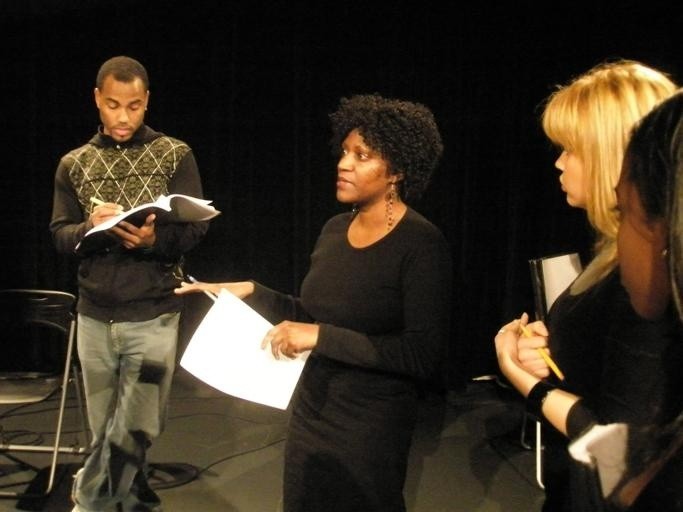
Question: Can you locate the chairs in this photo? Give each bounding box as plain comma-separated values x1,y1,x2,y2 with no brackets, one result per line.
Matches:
0,290,92,498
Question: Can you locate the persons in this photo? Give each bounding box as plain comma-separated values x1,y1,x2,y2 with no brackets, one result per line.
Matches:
561,87,681,510
172,92,452,512
46,56,209,511
493,56,681,511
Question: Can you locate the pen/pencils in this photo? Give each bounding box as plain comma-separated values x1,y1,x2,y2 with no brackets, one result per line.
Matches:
520,324,565,382
187,274,216,303
90,196,124,215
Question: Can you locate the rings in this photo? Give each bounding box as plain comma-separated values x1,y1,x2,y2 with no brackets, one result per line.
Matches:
498,327,504,334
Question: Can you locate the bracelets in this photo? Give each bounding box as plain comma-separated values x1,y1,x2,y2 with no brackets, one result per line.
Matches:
526,380,553,419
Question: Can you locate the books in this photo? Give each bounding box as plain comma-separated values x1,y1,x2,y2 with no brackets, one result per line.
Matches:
71,193,224,260
529,251,584,320
